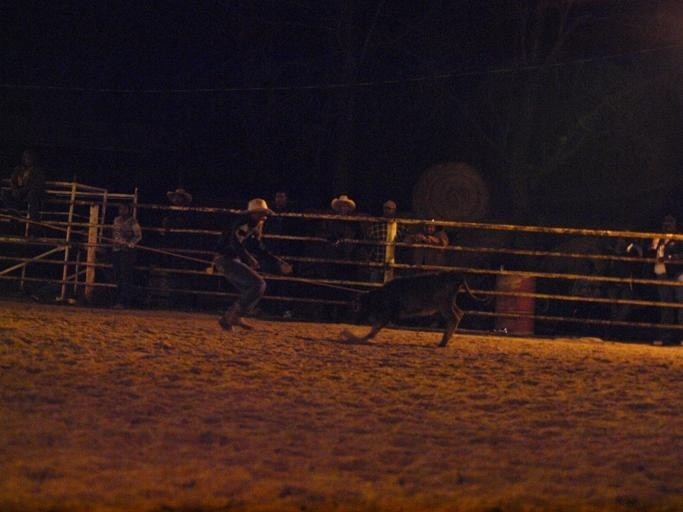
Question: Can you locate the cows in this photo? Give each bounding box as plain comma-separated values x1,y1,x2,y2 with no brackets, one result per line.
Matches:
337,267,496,347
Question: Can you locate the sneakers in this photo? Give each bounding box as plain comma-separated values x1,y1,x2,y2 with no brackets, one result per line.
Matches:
217,315,255,333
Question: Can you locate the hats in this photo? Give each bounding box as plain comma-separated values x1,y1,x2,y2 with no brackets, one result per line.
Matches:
239,198,275,217
330,194,357,214
166,188,192,206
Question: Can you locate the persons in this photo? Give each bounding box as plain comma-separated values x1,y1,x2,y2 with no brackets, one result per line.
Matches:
9,149,46,233
542,214,682,347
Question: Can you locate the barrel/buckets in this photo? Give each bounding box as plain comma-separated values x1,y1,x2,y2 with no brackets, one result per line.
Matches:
496,273,536,335
